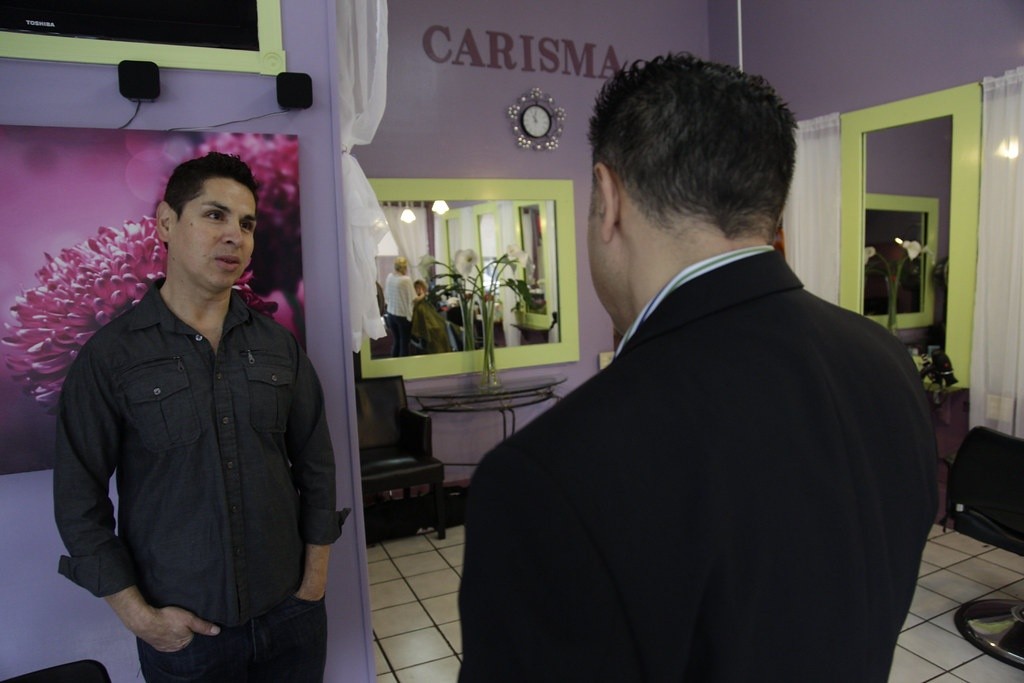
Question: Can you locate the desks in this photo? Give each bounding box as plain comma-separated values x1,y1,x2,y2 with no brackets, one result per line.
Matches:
406,374,568,467
928,386,967,533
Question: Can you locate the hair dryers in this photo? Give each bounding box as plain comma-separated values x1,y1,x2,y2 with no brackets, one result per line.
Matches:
918,345,958,389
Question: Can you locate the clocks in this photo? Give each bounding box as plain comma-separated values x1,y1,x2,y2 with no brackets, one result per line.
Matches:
507,88,566,151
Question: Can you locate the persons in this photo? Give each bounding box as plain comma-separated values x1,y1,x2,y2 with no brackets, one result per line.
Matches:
383,256,452,357
52,150,350,683
457,55,941,683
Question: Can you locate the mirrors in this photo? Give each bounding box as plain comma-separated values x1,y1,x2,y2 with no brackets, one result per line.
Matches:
362,176,580,376
471,204,503,320
838,84,982,388
512,199,550,329
863,193,939,330
443,208,463,285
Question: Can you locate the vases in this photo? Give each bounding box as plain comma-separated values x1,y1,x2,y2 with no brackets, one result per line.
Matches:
458,294,476,351
885,276,901,335
478,297,499,388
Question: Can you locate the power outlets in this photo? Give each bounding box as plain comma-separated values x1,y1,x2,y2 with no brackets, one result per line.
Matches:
598,351,615,370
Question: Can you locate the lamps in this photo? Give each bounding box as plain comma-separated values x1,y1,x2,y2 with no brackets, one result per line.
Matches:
400,200,416,223
431,200,449,214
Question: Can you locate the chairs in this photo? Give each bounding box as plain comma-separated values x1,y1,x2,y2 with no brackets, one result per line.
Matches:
355,375,446,550
942,425,1024,669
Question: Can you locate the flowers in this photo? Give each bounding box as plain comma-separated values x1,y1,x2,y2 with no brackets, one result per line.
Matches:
456,246,545,309
416,255,489,310
888,237,925,283
